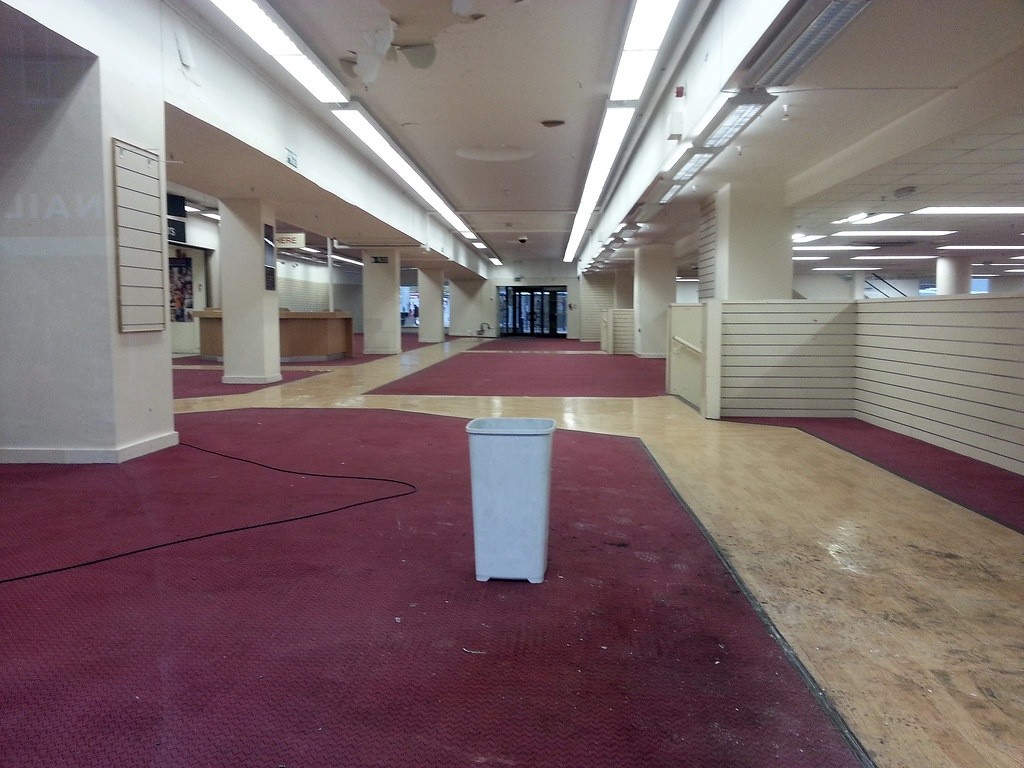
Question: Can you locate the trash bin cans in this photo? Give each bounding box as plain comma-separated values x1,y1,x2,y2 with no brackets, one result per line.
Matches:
465,417,558,584
